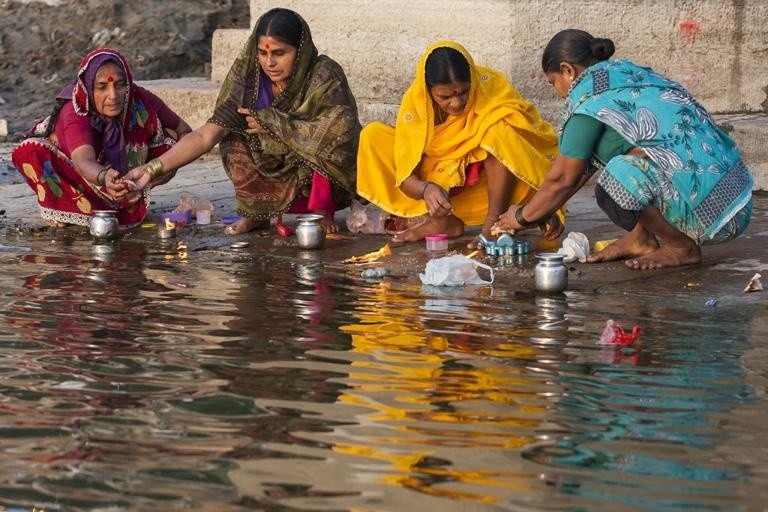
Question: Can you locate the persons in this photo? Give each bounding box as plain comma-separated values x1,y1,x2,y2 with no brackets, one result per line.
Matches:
171,238,358,503
3,233,166,494
358,45,565,251
511,284,764,500
11,47,190,227
491,30,751,269
334,244,545,510
116,8,365,234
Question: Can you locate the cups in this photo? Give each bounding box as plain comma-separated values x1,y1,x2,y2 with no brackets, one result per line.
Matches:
424,233,449,251
196,210,210,224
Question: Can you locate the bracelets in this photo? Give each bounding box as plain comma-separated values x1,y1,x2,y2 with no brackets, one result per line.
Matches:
416,180,432,199
96,165,112,187
144,158,162,176
514,205,539,226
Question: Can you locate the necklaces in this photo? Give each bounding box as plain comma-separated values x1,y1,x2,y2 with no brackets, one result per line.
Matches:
276,82,284,92
436,105,444,125
